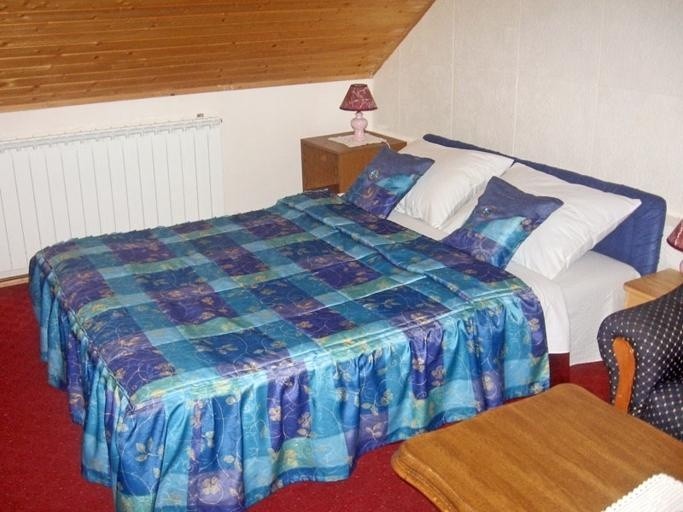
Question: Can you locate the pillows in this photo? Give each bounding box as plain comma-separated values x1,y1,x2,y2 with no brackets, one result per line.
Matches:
439,176,564,269
340,146,434,220
439,160,641,281
394,136,514,229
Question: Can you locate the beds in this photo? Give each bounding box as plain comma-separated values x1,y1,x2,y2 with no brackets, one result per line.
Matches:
30,134,667,512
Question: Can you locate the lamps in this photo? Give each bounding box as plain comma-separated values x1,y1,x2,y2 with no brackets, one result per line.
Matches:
340,84,377,139
667,218,683,271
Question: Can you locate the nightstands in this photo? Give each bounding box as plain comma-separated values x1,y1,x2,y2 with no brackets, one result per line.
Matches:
301,131,407,194
625,269,683,307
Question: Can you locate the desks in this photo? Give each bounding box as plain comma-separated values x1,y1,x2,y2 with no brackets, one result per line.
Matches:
392,382,682,511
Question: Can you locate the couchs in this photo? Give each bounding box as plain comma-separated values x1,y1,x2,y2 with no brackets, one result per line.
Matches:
596,283,682,440
